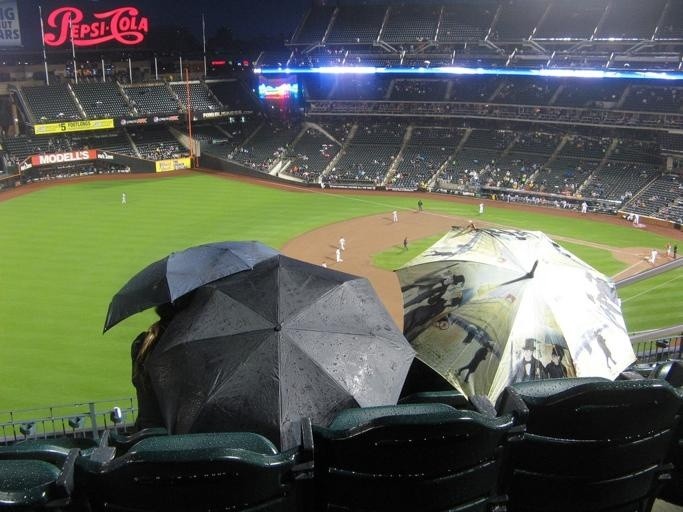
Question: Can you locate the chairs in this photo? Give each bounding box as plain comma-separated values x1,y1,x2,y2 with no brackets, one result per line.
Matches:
0,355,683,512
0,0,683,231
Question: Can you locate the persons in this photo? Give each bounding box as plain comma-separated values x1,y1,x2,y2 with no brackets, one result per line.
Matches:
650,249,657,265
664,240,671,256
424,60,432,67
673,245,678,258
287,117,467,144
392,198,424,222
65,59,114,77
2,153,19,174
95,100,103,105
630,85,680,106
26,134,88,154
350,77,433,95
500,125,652,161
403,237,409,251
121,192,127,208
321,261,327,267
38,162,131,180
130,295,190,428
622,165,683,222
227,144,435,192
310,102,489,116
276,47,362,66
435,157,620,214
512,228,626,381
336,248,342,263
208,104,216,110
480,80,555,100
338,236,345,250
532,107,676,125
127,131,183,160
39,111,154,120
128,99,141,108
401,273,494,384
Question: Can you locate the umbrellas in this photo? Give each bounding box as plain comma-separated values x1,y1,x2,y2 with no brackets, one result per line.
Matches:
103,240,280,336
143,254,417,453
393,223,638,399
